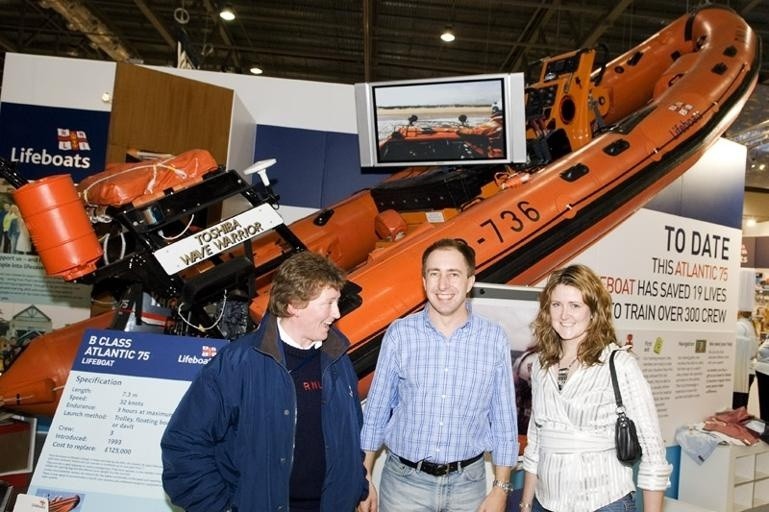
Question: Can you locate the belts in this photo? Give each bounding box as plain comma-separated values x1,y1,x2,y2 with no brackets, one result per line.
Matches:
398,452,485,477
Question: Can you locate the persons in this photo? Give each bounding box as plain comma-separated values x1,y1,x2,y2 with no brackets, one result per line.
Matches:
352,238,522,512
738,311,768,442
519,266,674,512
159,251,369,512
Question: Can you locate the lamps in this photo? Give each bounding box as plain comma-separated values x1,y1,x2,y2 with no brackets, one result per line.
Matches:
219,2,235,21
440,18,456,42
249,58,263,75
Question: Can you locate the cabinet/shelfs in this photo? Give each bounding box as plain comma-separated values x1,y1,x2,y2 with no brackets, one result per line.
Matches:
678,439,769,511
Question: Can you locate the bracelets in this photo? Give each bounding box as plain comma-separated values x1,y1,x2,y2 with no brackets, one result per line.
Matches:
520,501,534,510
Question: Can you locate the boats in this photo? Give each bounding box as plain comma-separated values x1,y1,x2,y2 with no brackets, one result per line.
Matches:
0,5,763,415
379,115,490,160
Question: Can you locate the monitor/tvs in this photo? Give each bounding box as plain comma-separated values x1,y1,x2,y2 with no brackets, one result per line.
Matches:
354,72,527,174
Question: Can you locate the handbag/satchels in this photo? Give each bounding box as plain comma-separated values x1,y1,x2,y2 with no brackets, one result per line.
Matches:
616,412,643,464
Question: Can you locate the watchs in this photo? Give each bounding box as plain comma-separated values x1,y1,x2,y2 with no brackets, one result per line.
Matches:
493,480,515,497
